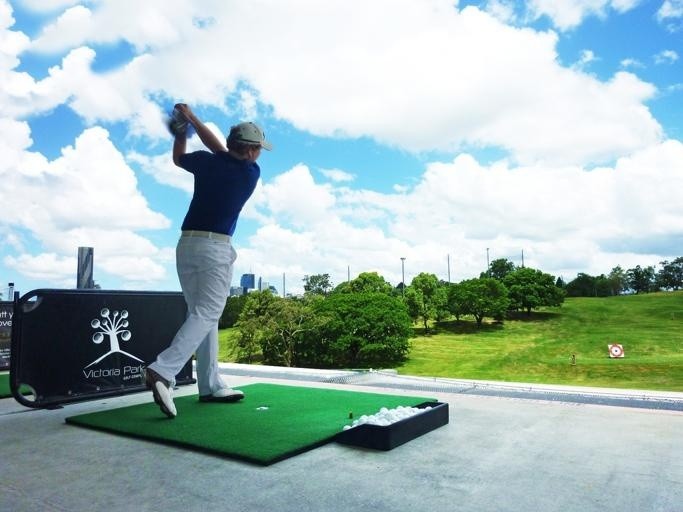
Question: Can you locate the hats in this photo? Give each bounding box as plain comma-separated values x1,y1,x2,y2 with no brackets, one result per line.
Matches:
230,121,272,151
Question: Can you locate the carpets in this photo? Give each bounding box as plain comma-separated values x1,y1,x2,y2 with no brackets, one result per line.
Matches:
65,383,437,467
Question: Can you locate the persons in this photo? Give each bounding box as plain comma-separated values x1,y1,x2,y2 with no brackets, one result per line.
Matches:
144,102,275,418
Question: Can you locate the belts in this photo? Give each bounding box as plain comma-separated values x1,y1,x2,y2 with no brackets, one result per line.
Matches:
181,230,232,244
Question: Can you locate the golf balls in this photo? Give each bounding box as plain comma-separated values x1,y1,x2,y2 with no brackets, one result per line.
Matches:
342,405,432,431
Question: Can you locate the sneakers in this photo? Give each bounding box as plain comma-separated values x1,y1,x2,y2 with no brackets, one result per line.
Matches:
198,385,245,402
139,366,178,420
